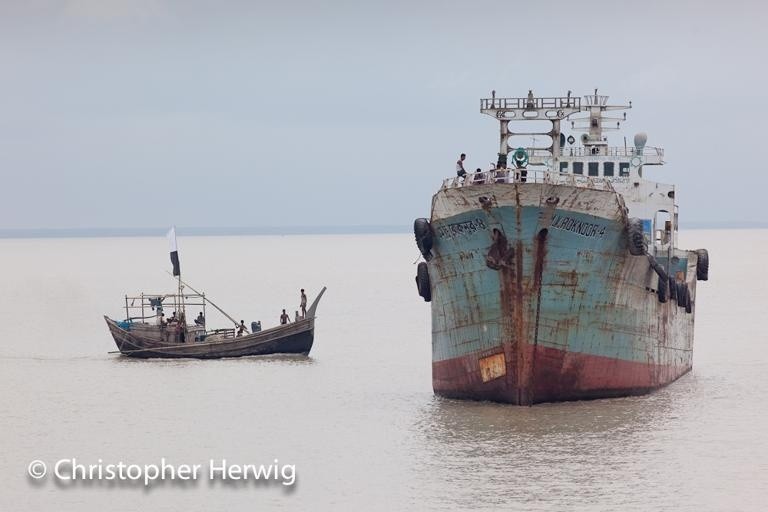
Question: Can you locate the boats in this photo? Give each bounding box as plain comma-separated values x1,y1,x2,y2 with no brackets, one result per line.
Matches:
417,93,713,405
102,286,327,359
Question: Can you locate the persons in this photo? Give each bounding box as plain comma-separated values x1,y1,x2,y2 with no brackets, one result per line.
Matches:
300,289,307,318
159,312,187,342
236,320,247,337
474,168,485,184
512,148,528,181
195,312,204,325
280,309,291,325
457,153,466,178
